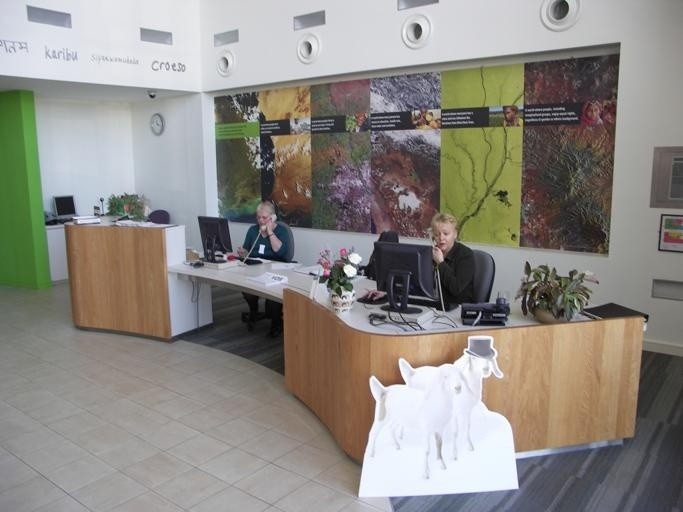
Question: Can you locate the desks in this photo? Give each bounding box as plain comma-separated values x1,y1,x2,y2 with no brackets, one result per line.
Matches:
43,214,116,282
61,214,210,343
168,250,371,306
281,287,647,464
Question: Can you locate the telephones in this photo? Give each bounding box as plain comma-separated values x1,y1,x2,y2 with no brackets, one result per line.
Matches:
260,215,277,232
428,228,435,247
461,292,509,325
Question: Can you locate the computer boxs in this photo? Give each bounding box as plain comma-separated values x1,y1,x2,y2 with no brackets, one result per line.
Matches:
195,257,241,270
370,302,436,326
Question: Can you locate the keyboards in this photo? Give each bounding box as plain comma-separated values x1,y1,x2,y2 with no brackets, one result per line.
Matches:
394,295,438,308
235,257,262,265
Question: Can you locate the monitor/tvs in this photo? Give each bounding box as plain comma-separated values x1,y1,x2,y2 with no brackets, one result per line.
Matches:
373,242,433,314
53,195,77,219
197,216,232,263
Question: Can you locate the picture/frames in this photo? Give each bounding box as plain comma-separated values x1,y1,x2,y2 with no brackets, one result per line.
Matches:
657,214,682,252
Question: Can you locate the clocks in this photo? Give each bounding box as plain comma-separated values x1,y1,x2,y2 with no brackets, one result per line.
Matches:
150,112,165,136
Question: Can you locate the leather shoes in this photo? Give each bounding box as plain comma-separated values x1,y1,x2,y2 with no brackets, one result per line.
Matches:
248,312,258,331
267,319,282,338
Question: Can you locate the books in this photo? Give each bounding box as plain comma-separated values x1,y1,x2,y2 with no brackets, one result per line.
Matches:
71,215,101,225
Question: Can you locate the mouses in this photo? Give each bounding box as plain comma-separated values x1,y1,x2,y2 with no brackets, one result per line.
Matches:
363,293,376,302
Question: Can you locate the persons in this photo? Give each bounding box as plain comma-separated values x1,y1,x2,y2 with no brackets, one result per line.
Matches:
364,213,475,319
290,115,302,135
500,105,523,126
350,114,368,132
410,104,437,129
237,201,294,333
579,99,604,125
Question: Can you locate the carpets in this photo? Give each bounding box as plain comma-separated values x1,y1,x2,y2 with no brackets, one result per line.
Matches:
175,282,683,511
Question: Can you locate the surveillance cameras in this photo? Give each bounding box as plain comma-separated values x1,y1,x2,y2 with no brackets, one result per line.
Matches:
148,91,156,98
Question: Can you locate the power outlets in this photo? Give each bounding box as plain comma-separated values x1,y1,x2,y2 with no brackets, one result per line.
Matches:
96,194,104,203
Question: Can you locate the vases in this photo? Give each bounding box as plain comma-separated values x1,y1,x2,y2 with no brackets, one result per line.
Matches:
328,292,354,314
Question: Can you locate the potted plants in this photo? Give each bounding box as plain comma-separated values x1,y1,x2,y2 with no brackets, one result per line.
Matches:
513,260,600,327
107,194,147,221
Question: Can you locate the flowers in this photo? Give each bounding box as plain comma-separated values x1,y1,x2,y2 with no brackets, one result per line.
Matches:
316,247,362,297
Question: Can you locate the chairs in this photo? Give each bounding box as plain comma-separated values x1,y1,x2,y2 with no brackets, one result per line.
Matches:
347,229,399,294
239,220,296,339
435,249,496,313
145,209,170,228
